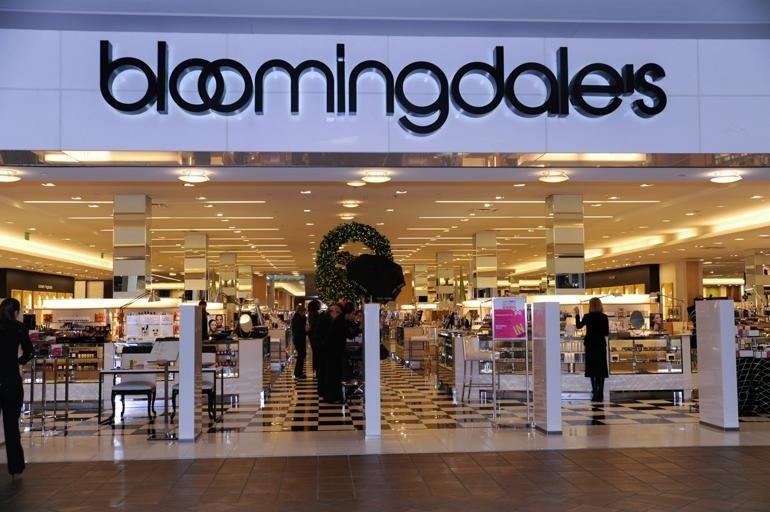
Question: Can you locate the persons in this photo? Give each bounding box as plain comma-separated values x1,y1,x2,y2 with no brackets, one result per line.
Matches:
307,298,364,403
250,309,257,317
199,300,210,340
575,296,611,403
291,304,307,379
209,319,218,334
651,314,662,330
1,297,37,480
215,315,225,333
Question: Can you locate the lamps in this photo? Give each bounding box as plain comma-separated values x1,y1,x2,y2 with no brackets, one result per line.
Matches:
536,167,571,185
361,170,392,184
0,166,21,185
177,167,213,183
708,168,744,185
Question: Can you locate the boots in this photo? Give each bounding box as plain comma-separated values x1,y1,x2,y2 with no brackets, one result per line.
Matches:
591,377,605,402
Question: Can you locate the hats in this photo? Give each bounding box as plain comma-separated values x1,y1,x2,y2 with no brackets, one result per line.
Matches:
330,304,343,313
